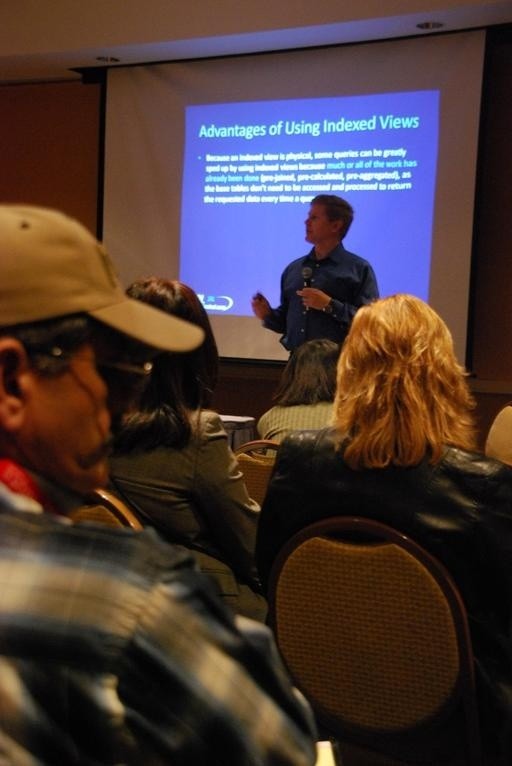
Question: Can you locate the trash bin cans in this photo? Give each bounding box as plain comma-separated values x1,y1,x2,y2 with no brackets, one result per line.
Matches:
322,297,336,314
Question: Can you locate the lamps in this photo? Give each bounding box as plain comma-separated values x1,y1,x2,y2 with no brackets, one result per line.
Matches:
266,516,482,766
235,439,279,504
65,488,151,530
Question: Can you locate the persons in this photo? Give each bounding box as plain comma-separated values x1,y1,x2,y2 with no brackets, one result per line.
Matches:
252,193,381,351
253,288,512,766
107,275,272,626
2,204,319,766
254,338,343,462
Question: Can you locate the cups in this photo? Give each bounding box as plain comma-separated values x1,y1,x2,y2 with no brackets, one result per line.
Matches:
36,348,156,395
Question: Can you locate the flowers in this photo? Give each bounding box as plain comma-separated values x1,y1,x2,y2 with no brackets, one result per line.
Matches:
302,265,314,313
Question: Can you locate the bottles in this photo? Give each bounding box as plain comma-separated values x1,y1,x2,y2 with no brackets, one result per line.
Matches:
0,202,208,354
